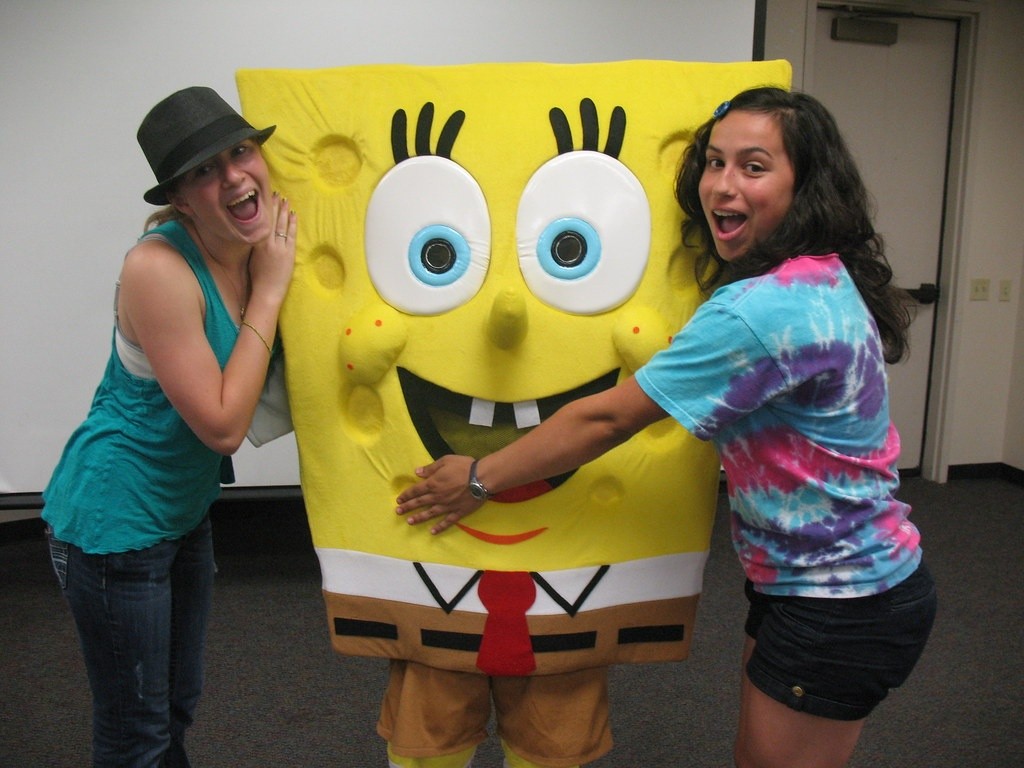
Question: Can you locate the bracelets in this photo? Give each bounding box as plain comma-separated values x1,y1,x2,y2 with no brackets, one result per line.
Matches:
242,321,271,353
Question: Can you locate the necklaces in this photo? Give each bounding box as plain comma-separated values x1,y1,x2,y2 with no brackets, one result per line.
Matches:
217,262,247,317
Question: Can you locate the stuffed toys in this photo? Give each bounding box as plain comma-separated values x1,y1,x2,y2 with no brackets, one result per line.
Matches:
238,59,797,768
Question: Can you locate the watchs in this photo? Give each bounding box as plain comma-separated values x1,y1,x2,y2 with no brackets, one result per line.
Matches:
468,459,493,501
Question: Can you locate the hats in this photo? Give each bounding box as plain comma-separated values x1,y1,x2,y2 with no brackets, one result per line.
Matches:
137,85,277,205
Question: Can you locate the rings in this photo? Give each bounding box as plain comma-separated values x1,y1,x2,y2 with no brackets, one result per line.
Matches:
275,233,286,238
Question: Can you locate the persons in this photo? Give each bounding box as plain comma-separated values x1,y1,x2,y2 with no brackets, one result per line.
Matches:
398,87,939,768
40,87,298,768
